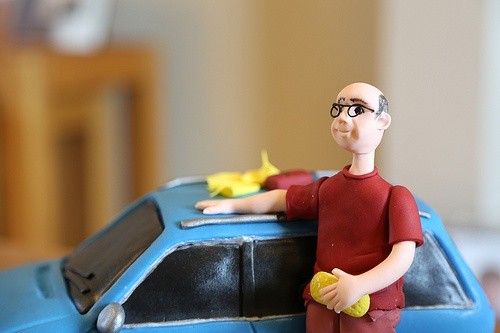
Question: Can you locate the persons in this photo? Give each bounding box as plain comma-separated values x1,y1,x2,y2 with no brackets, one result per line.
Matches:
193,82,423,333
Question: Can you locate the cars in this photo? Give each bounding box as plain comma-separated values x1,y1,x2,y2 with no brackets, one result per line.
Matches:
0,169,497,333
